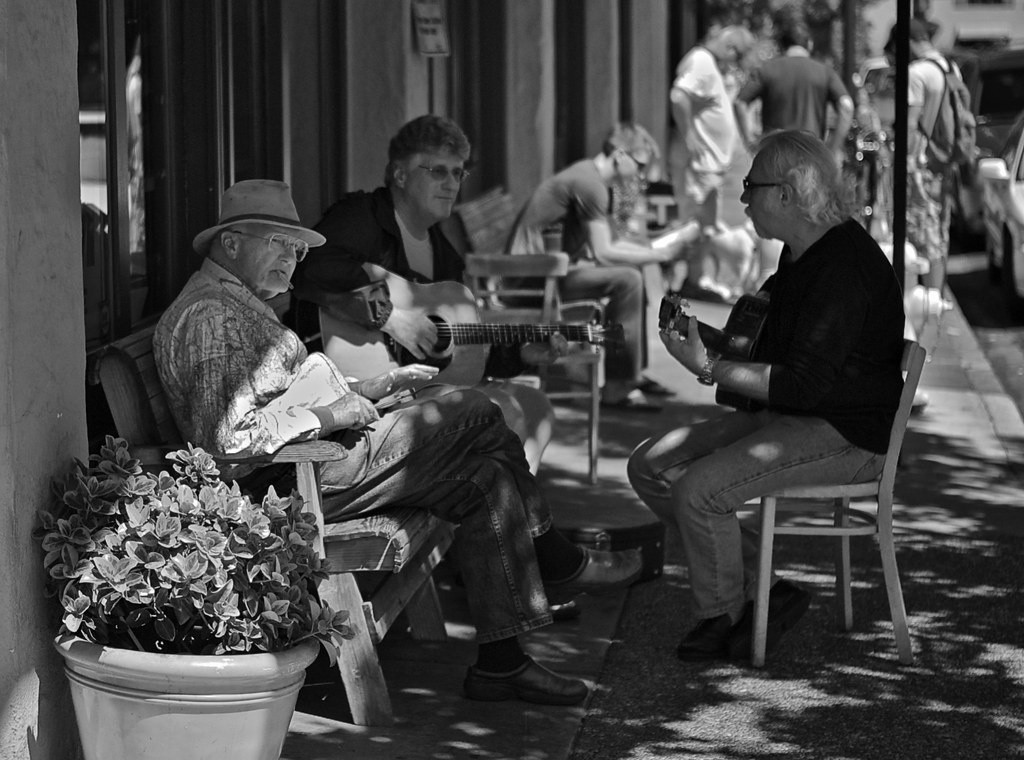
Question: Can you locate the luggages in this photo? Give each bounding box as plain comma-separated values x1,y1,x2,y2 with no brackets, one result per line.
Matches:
733,45,743,61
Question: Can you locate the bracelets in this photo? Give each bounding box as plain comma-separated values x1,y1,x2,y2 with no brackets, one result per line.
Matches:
357,379,380,405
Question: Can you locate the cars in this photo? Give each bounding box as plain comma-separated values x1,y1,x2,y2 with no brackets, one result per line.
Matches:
851,25,1024,300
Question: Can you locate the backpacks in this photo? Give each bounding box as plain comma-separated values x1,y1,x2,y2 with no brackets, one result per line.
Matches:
908,56,977,174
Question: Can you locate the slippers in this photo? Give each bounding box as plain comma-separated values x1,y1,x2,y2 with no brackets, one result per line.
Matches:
636,375,677,397
601,392,663,413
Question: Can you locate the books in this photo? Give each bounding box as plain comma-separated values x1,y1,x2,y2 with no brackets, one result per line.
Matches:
650,219,699,250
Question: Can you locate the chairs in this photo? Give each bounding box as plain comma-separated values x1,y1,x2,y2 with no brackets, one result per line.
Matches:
736,338,925,671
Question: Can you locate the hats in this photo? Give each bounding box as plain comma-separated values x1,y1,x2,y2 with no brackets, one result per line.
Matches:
192,178,327,257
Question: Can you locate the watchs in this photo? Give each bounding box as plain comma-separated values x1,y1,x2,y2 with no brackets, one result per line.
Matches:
697,354,721,386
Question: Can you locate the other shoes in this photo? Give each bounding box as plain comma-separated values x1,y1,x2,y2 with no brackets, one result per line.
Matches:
458,651,589,708
541,545,644,591
676,577,812,663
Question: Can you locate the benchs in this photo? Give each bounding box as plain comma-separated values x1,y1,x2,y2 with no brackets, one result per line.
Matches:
88,187,677,726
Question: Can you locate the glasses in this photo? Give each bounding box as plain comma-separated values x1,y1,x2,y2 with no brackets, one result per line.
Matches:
628,151,646,171
232,230,309,262
418,163,470,185
742,179,782,192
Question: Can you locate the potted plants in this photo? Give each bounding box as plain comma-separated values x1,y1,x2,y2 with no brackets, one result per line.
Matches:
30,435,354,759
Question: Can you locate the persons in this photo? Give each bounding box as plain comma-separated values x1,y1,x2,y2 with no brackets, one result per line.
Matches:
733,17,854,292
502,121,701,418
506,123,700,413
881,20,977,316
627,127,905,668
669,25,741,304
289,117,563,587
153,179,591,704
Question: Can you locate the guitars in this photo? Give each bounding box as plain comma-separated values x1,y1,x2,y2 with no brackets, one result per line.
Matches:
658,268,778,416
319,261,627,410
